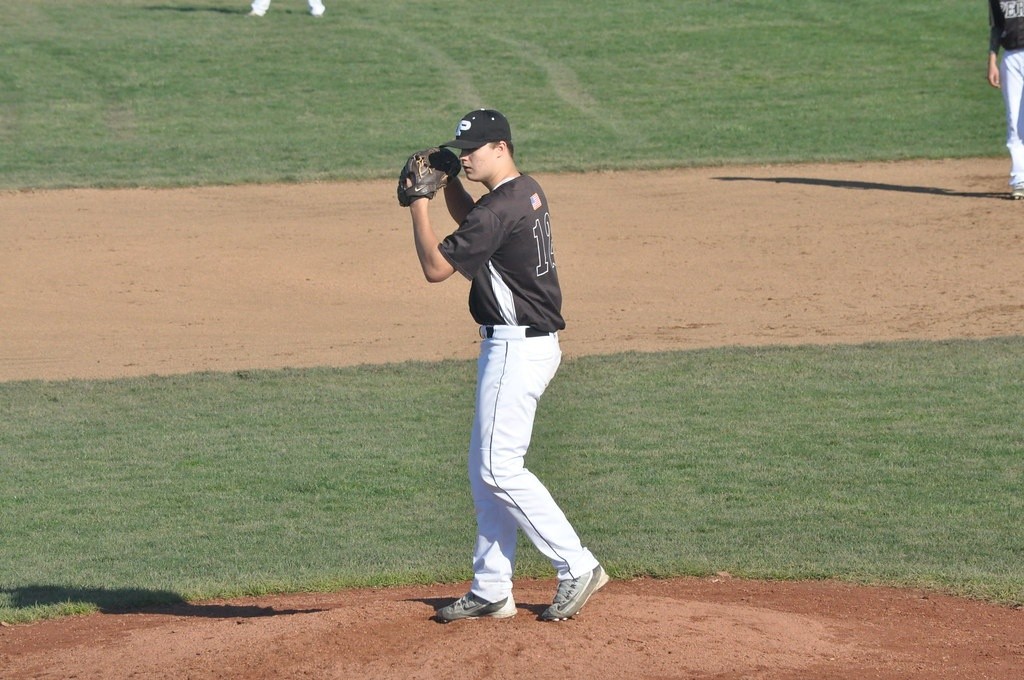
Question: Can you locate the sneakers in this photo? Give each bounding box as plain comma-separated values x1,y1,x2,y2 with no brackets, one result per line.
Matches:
437,591,518,623
542,565,610,621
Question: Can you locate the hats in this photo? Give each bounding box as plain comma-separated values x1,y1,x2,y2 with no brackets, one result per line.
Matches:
438,108,511,149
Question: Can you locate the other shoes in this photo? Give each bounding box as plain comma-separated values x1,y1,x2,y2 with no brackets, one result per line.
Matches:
249,10,258,16
1011,189,1024,200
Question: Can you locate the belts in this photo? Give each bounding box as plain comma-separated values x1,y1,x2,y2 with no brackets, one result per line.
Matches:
479,326,549,339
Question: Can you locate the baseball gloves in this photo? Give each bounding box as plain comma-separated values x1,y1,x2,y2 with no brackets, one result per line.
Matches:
397,146,461,207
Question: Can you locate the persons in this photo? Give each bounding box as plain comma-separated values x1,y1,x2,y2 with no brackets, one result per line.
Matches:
398,110,611,624
987,0,1024,199
249,0,326,18
